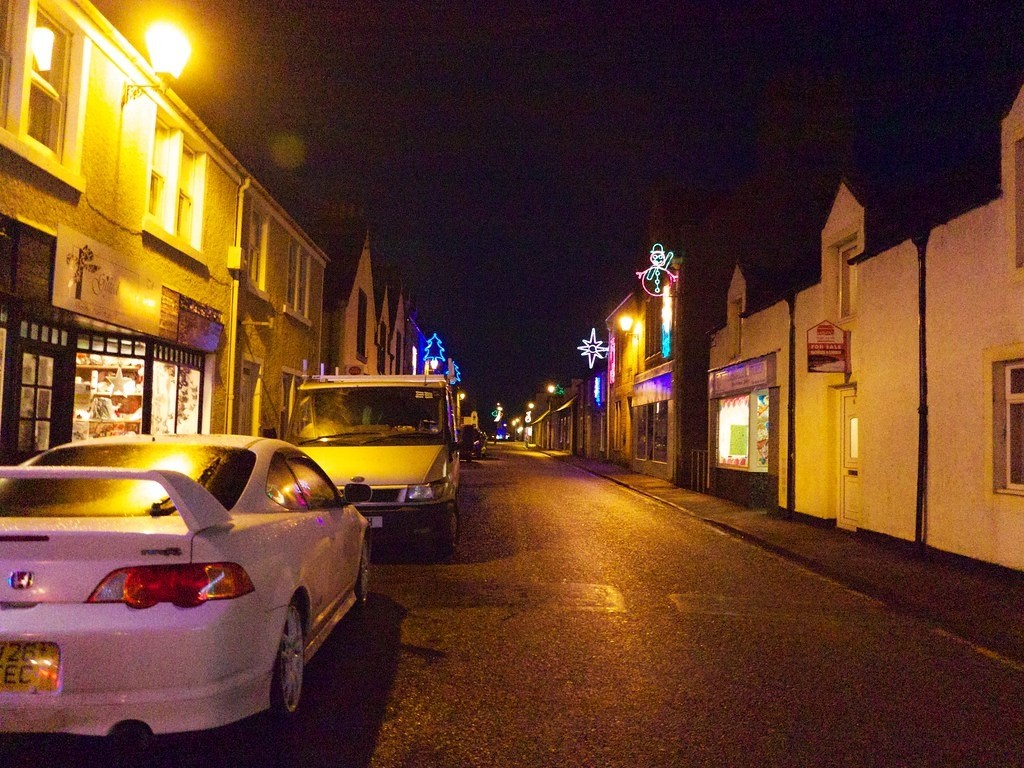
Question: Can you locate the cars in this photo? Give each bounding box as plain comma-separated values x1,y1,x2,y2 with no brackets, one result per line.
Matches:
0,431,371,751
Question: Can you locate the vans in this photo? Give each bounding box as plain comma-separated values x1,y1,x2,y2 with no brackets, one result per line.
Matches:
288,372,460,556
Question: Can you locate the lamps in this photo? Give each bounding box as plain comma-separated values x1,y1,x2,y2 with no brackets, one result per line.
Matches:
121,21,193,107
